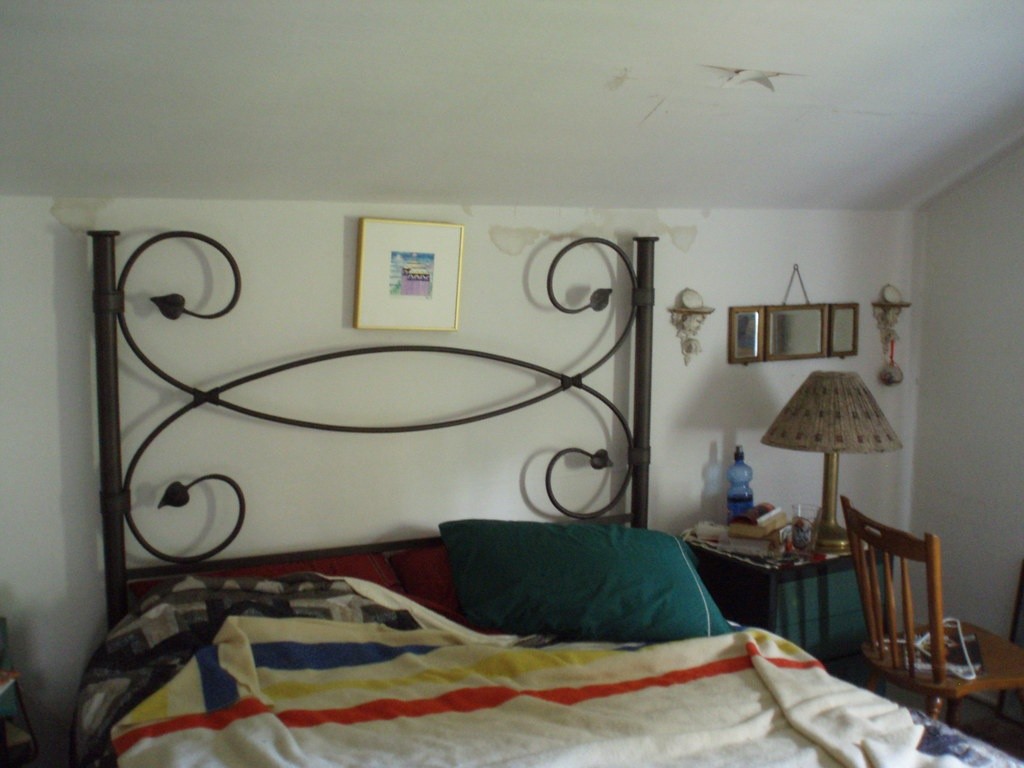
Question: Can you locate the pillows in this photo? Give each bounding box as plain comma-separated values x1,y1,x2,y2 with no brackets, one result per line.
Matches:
129,553,404,600
439,519,732,639
390,542,461,619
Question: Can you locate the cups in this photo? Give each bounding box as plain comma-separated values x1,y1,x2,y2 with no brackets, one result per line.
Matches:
791,503,822,557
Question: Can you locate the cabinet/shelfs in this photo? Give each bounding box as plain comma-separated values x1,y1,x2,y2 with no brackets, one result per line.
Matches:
682,532,893,700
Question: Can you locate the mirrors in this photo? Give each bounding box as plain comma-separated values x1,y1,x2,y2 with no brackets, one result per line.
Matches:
728,302,859,363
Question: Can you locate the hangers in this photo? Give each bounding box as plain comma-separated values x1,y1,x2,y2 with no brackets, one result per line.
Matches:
875,617,976,682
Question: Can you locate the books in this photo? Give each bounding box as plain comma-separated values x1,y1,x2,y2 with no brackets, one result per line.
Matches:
874,631,984,678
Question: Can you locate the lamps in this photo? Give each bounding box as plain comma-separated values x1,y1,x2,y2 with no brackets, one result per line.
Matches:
669,288,716,365
761,371,903,548
872,284,911,384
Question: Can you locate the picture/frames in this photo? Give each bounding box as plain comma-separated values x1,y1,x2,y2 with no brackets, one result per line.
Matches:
354,219,463,332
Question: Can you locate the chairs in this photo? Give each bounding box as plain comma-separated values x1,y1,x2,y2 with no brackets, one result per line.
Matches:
840,496,1024,752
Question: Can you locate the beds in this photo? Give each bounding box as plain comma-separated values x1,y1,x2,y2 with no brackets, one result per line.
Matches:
69,232,1024,768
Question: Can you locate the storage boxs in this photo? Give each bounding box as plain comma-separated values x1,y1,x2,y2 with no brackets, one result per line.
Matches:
729,507,786,540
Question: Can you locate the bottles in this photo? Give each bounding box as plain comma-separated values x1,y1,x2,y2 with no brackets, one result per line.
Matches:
726,444,758,527
